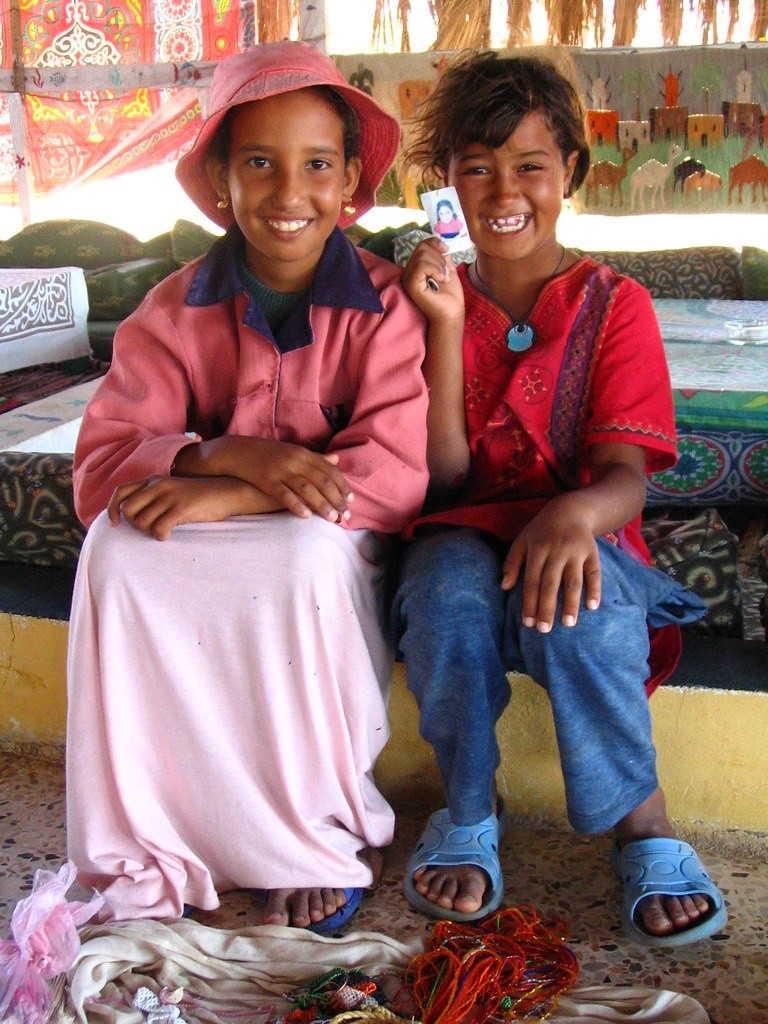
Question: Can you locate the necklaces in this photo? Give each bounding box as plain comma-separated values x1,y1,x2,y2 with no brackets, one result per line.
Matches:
475,244,565,352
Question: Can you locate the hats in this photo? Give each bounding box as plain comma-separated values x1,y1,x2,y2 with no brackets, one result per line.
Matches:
176,46,399,231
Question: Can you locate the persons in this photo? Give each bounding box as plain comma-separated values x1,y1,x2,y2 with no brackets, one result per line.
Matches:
390,49,731,950
434,199,464,239
63,41,430,934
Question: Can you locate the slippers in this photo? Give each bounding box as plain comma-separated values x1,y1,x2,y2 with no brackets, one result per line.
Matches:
404,790,506,920
610,837,727,948
263,874,361,933
